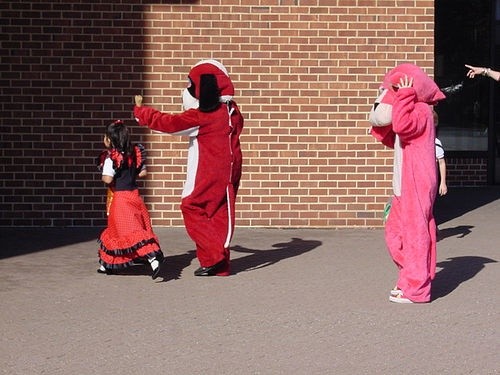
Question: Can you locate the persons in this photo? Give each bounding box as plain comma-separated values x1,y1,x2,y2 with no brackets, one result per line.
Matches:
465,64,500,82
434,137,448,196
97,120,165,280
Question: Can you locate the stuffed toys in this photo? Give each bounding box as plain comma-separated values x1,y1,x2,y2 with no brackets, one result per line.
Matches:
134,59,244,277
367,64,446,304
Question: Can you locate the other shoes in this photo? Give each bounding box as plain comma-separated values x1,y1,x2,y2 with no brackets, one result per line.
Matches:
97,264,113,275
148,253,168,280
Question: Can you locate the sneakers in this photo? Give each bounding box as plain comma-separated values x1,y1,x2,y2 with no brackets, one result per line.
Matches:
388,291,414,304
390,285,402,295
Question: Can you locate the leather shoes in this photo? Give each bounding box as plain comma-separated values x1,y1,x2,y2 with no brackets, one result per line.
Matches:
193,260,227,276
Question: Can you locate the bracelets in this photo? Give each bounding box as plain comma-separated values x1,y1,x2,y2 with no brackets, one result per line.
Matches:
482,67,490,77
